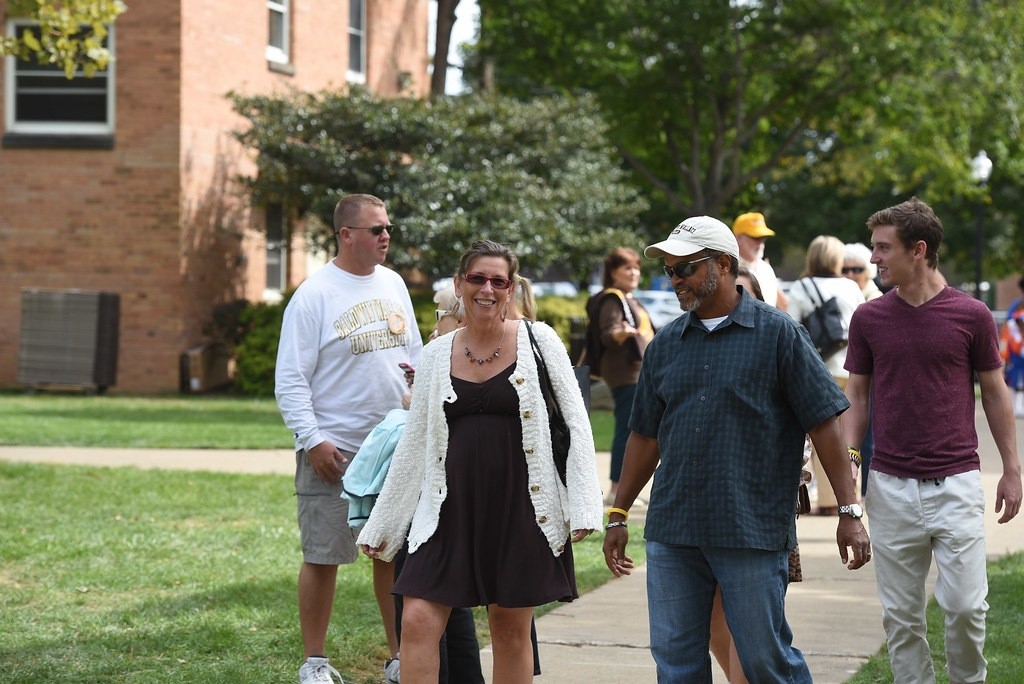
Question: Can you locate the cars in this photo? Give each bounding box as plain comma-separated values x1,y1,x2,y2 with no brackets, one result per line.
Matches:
630,289,683,334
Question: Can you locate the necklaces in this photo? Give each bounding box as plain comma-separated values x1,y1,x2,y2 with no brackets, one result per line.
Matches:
464,326,505,364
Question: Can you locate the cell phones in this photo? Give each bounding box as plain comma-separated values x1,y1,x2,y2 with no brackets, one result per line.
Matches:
398,362,415,383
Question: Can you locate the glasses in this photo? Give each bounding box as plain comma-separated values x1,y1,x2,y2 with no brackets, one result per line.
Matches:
436,310,452,322
842,267,863,276
337,225,395,235
663,255,711,279
464,273,512,289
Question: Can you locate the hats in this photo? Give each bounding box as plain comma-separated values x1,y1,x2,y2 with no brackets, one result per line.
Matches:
644,216,739,262
734,211,776,237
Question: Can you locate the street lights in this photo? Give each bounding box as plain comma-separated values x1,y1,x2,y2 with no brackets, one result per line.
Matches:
969,148,993,384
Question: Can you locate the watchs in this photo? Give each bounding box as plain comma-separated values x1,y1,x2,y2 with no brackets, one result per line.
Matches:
838,503,863,519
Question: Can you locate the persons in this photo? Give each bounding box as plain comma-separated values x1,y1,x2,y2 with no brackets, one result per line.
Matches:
841,197,1023,684
355,241,603,684
586,247,658,506
273,194,424,684
602,216,871,684
999,277,1024,420
732,212,884,517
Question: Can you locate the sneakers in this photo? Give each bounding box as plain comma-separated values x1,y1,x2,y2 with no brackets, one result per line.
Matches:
384,653,401,684
298,656,344,684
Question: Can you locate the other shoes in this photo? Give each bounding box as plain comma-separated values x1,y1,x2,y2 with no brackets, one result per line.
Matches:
820,506,839,515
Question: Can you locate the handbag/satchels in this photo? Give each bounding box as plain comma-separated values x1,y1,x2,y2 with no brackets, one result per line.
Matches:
522,319,591,488
800,275,849,362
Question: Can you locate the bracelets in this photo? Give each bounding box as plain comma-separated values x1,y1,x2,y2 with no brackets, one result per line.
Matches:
606,508,628,521
849,453,860,468
848,450,861,463
605,522,627,530
